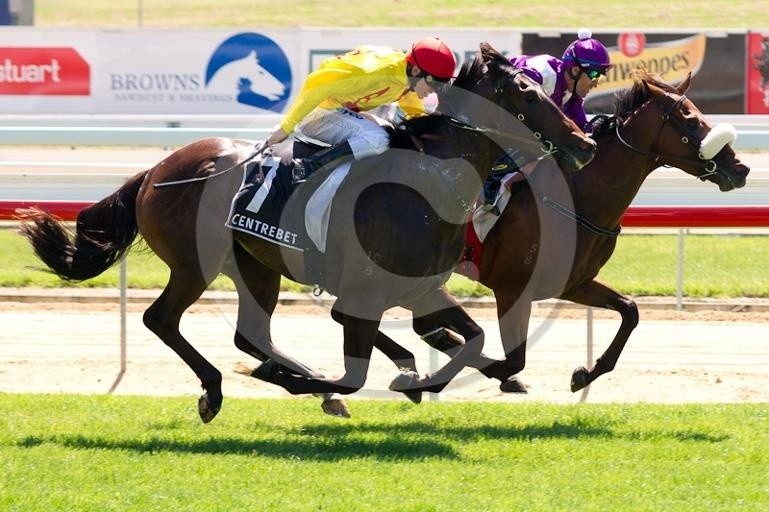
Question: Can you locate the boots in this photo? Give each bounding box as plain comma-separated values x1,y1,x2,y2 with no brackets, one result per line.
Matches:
292,140,354,180
481,146,538,215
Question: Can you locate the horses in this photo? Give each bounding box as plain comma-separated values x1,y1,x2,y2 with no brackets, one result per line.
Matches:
330,67,752,402
14,40,597,425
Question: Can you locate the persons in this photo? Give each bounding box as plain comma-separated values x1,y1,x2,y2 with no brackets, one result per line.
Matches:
258,34,456,186
461,35,615,225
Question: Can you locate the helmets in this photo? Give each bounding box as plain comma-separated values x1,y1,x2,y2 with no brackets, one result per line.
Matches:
407,37,456,82
562,29,615,70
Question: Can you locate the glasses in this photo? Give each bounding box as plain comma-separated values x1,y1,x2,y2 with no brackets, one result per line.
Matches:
572,49,601,82
412,47,444,88
